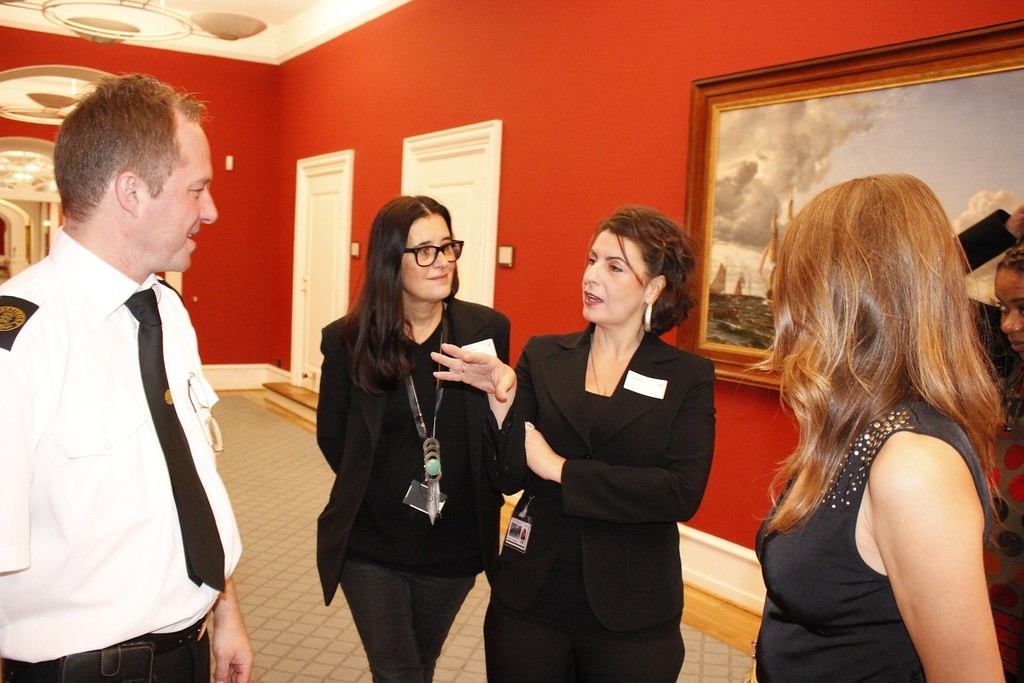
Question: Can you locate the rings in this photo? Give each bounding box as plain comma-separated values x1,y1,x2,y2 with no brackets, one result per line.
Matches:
460,363,464,372
526,421,534,431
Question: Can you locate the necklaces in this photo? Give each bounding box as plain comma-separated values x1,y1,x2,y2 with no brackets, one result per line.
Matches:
589,334,642,397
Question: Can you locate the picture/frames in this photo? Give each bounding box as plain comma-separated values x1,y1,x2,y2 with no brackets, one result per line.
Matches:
677,20,1024,432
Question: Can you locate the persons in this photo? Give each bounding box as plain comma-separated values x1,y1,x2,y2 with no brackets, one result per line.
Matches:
317,197,510,683
431,204,715,682
0,73,256,683
736,173,1005,683
957,204,1024,682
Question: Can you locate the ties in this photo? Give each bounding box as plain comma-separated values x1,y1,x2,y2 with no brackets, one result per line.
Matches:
124,283,228,593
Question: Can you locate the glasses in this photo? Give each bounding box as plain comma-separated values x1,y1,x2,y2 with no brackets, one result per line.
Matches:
401,237,466,267
